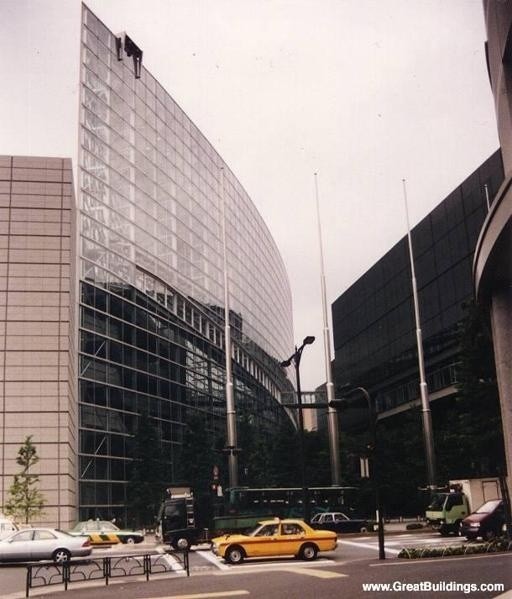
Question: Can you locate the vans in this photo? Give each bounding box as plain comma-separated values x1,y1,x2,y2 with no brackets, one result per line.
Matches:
460,500,507,542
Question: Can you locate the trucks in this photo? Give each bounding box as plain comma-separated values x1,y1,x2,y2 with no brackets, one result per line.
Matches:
426,477,504,537
158,487,361,552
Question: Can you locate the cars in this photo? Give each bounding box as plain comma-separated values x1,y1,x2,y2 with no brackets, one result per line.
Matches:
0,519,143,566
309,512,379,533
210,517,338,563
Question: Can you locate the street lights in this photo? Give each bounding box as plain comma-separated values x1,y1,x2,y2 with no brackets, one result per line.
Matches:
281,336,315,522
